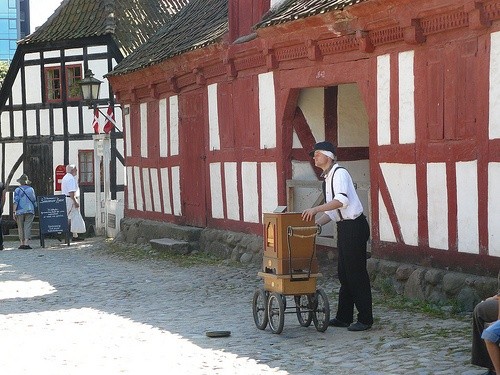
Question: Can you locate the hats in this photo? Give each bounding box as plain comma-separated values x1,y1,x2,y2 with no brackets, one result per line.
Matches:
16,173,32,185
309,141,336,156
66,165,76,172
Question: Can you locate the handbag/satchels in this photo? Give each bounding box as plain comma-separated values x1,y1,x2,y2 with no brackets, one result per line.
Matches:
34,201,39,216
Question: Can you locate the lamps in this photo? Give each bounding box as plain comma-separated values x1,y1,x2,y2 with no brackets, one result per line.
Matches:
77,69,130,117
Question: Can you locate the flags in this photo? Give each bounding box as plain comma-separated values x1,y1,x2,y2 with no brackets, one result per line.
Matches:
103,105,115,134
92,108,99,134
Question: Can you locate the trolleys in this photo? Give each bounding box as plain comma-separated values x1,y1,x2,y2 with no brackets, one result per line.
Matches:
251,206,330,334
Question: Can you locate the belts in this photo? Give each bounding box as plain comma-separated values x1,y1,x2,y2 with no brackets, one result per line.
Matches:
65,195,78,199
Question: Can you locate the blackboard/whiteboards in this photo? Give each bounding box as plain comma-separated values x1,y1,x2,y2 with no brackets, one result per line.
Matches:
37,195,69,234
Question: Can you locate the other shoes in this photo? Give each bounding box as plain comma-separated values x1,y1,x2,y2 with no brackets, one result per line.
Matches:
72,237,84,241
18,245,33,249
322,319,350,327
348,321,371,331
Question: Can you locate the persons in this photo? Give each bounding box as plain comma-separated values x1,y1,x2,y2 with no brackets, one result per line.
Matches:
0,181,6,251
58,164,85,242
13,174,38,249
472,293,500,375
301,141,373,331
